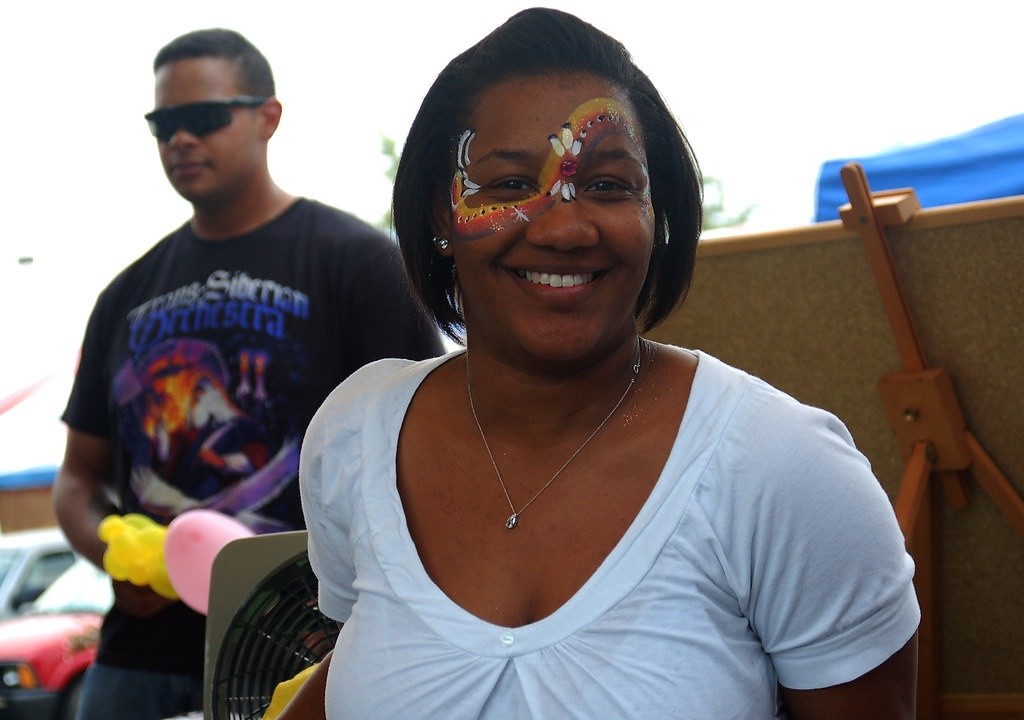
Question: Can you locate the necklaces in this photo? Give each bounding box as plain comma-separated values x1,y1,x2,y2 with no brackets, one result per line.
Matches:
466,336,641,530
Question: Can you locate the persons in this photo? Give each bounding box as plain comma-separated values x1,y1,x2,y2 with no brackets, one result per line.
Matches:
52,28,447,720
277,7,924,719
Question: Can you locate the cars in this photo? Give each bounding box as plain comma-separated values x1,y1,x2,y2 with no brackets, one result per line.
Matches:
0,527,80,633
0,556,116,720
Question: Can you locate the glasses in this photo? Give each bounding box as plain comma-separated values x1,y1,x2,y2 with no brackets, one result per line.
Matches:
144,94,268,142
